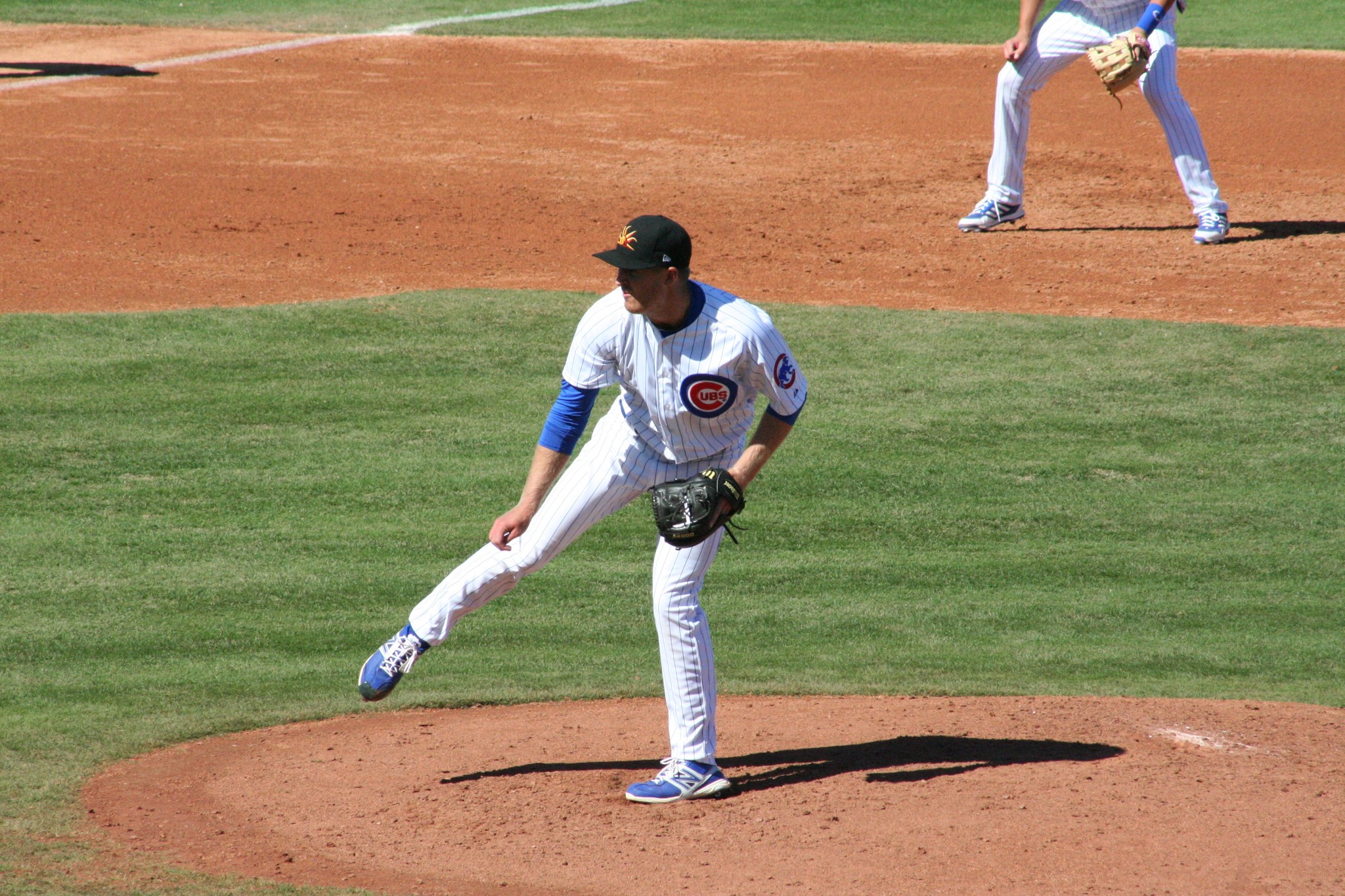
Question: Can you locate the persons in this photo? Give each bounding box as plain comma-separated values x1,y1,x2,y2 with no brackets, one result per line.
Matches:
358,216,808,804
959,0,1230,245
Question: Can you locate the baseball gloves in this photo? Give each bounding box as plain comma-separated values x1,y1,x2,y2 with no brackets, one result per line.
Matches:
652,466,746,548
1086,37,1149,96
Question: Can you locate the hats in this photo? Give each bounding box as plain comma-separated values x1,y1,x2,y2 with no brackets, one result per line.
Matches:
592,215,692,270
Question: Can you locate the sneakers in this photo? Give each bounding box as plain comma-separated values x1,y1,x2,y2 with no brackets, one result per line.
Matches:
359,624,432,704
625,757,732,804
958,196,1025,232
1192,208,1231,245
